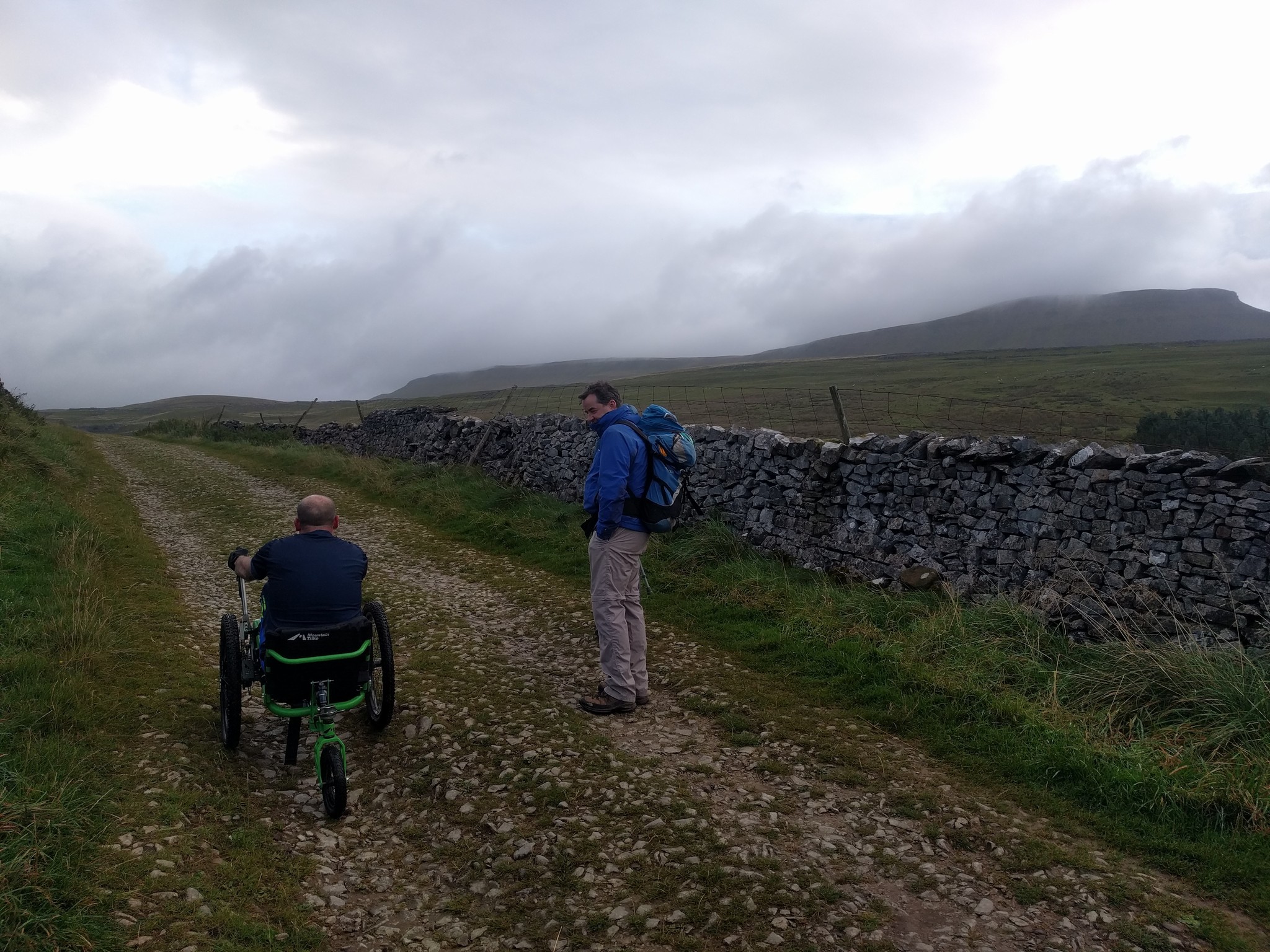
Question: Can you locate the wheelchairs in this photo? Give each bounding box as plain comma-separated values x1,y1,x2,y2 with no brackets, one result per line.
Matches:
217,544,398,821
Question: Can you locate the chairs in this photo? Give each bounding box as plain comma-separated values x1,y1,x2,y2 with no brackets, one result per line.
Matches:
265,616,371,694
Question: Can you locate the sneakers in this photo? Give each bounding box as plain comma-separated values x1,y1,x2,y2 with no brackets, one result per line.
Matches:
598,680,649,705
580,694,637,715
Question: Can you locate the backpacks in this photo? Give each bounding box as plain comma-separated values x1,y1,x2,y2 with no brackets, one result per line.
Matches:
610,404,697,534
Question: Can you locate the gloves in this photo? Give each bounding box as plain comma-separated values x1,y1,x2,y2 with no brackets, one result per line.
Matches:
227,546,249,571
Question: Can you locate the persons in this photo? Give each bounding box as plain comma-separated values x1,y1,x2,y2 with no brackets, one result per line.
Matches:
227,493,369,632
577,378,651,714
